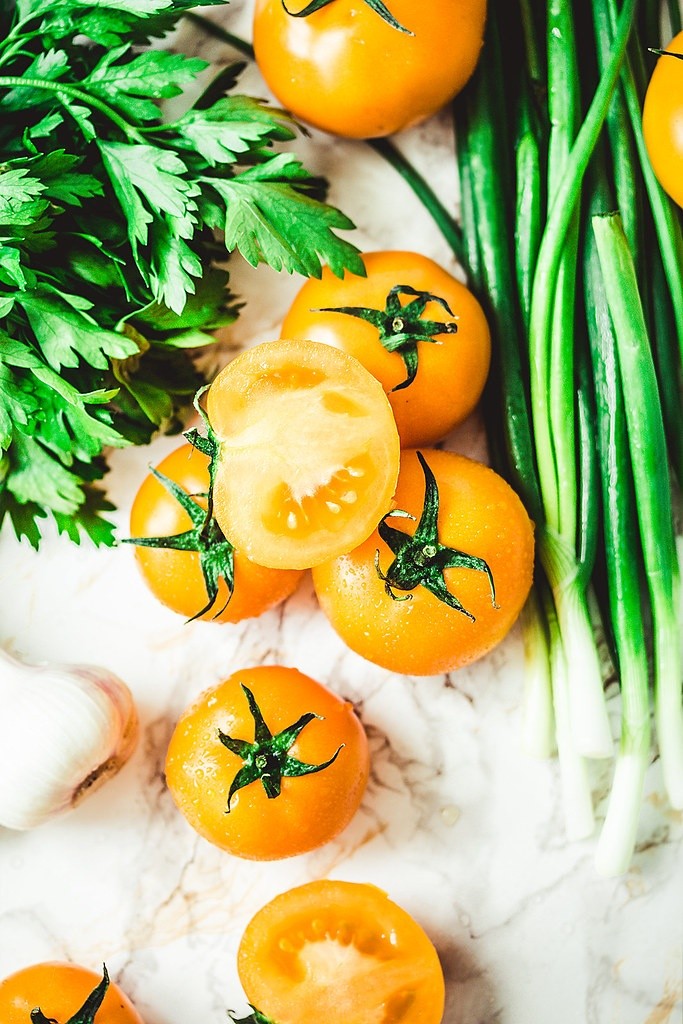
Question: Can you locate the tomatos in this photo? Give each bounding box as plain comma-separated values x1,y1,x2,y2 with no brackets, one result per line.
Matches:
228,879,444,1024
310,446,535,677
122,441,307,622
183,339,400,570
642,31,683,209
276,251,489,447
0,961,147,1024
251,0,487,140
165,665,371,861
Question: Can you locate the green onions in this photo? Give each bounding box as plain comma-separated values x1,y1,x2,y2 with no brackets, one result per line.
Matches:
171,0,683,876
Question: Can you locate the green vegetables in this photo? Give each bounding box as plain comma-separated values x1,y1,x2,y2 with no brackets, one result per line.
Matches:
0,0,366,555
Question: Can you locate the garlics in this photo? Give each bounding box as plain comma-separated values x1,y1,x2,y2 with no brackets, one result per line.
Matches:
0,646,140,832
